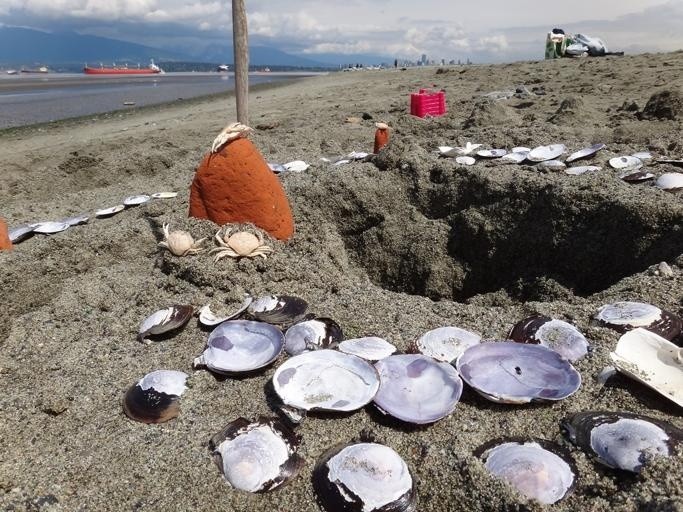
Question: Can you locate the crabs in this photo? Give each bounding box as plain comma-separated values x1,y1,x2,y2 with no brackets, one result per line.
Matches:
210,122,255,155
208,227,275,267
158,221,207,257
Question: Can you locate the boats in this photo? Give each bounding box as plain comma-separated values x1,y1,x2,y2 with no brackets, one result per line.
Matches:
5,64,63,75
82,58,164,74
217,65,228,71
263,65,271,72
